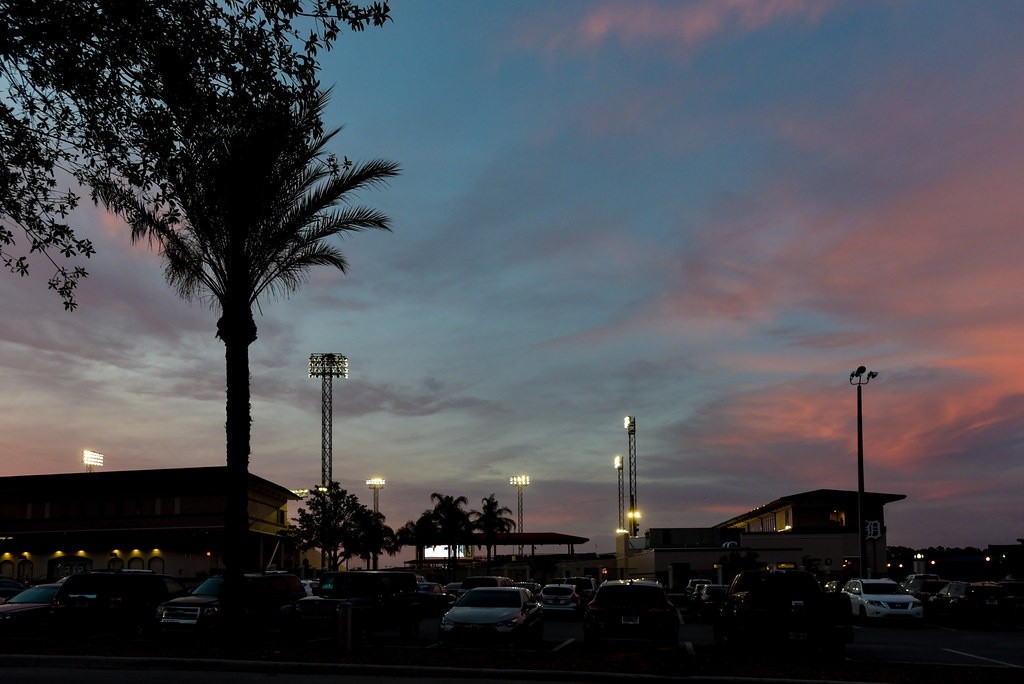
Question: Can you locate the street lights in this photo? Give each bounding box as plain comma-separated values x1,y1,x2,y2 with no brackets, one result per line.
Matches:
849,366,878,578
82,449,104,473
365,478,385,570
614,455,625,533
308,352,349,571
624,415,639,534
510,474,531,559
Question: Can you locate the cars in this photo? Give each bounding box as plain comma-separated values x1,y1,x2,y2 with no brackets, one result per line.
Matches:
300,571,729,624
534,584,581,623
583,578,682,646
711,569,855,661
436,586,542,648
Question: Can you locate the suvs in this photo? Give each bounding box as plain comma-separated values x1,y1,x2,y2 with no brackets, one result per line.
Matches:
0,568,307,661
289,572,430,651
840,578,924,627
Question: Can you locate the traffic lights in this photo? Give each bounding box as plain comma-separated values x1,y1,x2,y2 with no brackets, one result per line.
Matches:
601,568,609,581
